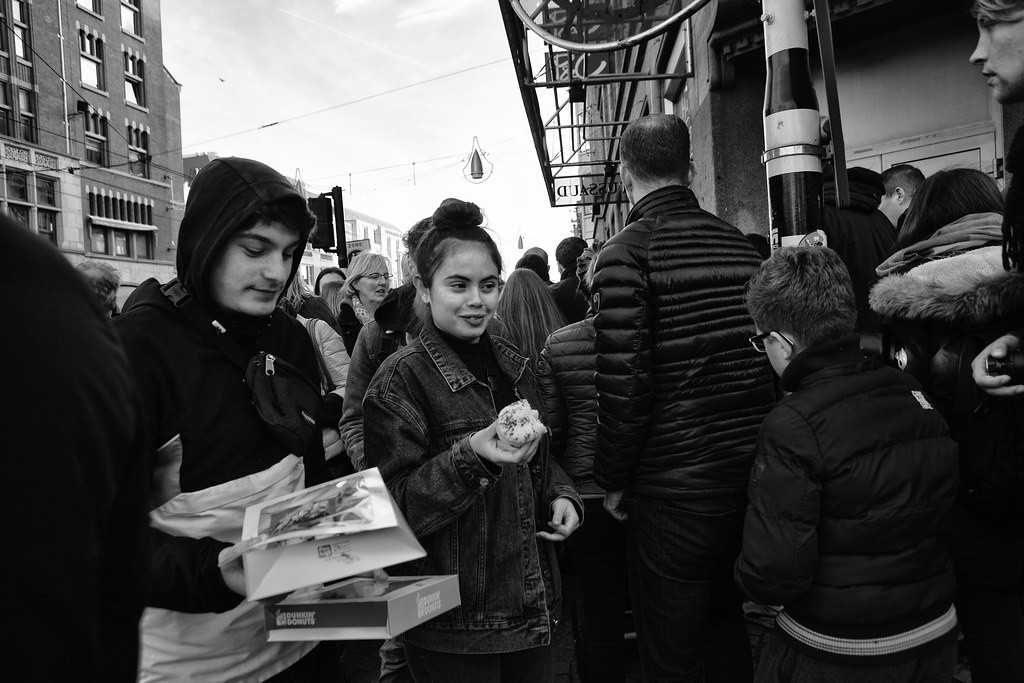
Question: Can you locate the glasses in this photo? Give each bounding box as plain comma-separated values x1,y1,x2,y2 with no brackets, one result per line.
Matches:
360,273,393,279
748,329,795,353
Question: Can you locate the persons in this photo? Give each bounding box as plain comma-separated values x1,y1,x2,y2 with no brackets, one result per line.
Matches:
117,158,390,682
361,202,584,683
869,167,1016,396
76,262,120,316
593,114,778,682
729,246,965,682
0,213,145,683
814,209,875,340
338,218,515,683
283,247,590,384
966,0,1023,418
278,297,351,479
537,242,624,683
810,162,895,272
743,234,770,261
878,165,924,230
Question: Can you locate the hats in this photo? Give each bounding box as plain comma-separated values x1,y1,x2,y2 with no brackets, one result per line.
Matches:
556,237,588,267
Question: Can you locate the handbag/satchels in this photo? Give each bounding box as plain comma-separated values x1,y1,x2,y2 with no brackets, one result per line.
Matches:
246,351,326,484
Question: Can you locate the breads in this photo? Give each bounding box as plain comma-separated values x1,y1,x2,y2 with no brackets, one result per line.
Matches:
496,398,549,449
